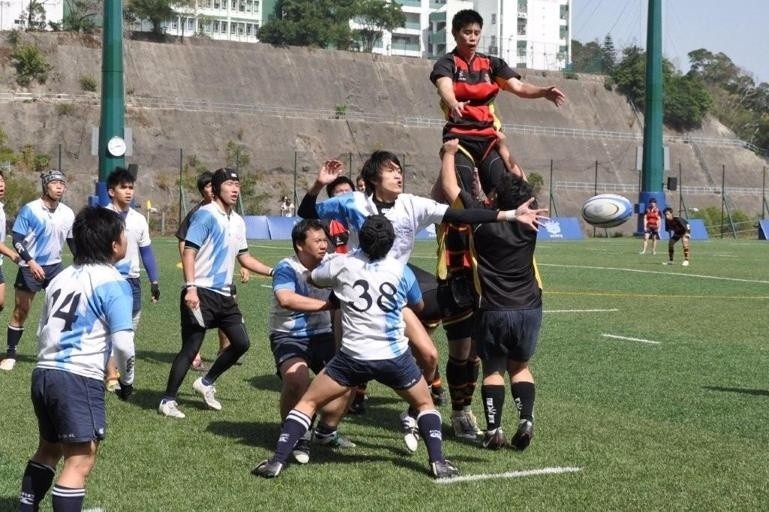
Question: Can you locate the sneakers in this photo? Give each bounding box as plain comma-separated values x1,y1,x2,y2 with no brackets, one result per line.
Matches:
313,429,356,448
192,377,221,410
252,457,283,476
661,260,674,265
681,260,689,266
158,398,185,418
1,352,19,371
350,392,366,414
399,411,534,479
293,429,312,464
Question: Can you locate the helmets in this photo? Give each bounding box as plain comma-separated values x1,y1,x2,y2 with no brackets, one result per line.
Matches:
40,169,66,195
360,215,394,255
211,167,239,195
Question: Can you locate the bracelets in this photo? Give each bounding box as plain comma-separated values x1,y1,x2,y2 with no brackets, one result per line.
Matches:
185,282,196,289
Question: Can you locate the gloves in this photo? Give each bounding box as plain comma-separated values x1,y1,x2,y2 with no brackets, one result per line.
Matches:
114,376,133,401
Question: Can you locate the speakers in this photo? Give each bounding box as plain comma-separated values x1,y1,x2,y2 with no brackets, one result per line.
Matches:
668,176,678,190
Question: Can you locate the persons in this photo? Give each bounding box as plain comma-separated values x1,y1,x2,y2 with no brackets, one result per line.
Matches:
175,169,251,372
0,171,20,315
280,195,294,217
428,9,566,198
104,167,161,393
638,197,662,255
0,169,78,372
661,207,692,266
157,168,275,419
12,206,136,512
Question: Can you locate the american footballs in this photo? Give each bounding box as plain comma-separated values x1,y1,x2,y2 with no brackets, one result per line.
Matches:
581,194,633,228
